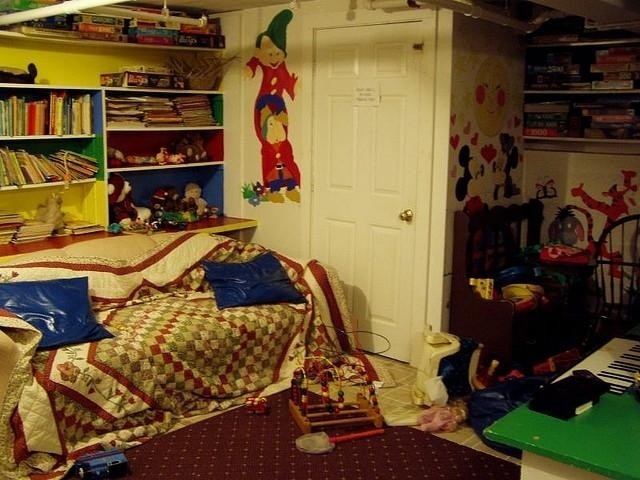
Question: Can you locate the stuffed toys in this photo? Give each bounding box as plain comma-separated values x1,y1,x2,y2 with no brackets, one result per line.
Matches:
108,131,220,234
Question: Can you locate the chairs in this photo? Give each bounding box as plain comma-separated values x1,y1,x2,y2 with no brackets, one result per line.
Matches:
595,214,639,343
448,198,543,367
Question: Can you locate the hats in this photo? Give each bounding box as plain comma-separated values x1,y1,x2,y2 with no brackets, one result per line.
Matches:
256,8,294,59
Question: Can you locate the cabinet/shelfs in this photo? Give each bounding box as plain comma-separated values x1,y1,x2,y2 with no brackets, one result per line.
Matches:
106,86,223,229
2,86,106,242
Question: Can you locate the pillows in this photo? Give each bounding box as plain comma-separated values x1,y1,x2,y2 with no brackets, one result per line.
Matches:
200,250,309,310
0,276,116,351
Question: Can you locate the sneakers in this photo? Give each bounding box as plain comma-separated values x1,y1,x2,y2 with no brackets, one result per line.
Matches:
268,188,302,203
260,105,289,128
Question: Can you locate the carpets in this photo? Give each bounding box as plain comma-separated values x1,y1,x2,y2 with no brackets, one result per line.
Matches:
0,386,521,480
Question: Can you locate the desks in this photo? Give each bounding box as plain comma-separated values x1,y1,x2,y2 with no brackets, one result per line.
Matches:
483,334,639,480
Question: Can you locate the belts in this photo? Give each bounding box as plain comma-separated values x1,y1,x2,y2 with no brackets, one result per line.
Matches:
274,163,286,170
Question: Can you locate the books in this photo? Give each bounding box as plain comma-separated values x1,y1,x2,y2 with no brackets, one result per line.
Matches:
0,91,107,246
105,92,220,128
20,13,226,49
522,47,640,138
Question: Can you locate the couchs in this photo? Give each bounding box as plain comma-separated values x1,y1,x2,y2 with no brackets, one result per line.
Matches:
0,231,361,478
